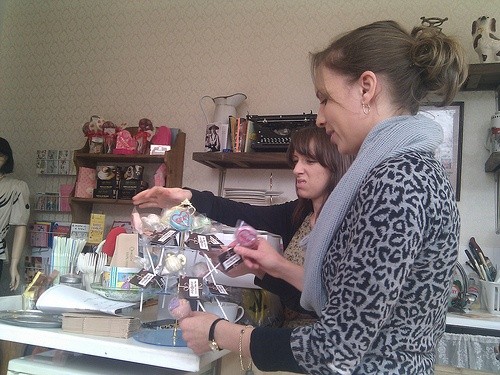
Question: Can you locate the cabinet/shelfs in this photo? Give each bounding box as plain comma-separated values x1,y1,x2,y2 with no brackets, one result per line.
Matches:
460,62,500,172
19,159,76,283
66,127,186,255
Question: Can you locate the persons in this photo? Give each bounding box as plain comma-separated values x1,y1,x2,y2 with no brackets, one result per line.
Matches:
0,138,30,290
179,21,468,375
131,127,354,327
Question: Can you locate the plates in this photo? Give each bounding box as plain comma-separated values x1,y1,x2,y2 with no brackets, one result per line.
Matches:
90,282,147,301
0,309,62,328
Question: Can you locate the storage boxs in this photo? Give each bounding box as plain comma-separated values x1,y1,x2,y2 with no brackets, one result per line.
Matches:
110,232,140,267
74,166,97,199
89,213,105,243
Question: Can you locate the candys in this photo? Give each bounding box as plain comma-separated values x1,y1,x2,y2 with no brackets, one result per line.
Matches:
192,262,209,278
168,297,193,319
236,225,259,248
130,205,212,235
166,253,186,274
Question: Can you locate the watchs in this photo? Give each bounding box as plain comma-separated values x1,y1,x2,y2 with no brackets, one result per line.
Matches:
209,317,229,352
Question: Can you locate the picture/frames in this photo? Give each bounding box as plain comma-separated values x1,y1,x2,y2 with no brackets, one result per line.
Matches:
417,101,464,201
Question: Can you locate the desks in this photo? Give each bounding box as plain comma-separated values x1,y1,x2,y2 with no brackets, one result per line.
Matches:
0,321,234,375
433,309,500,375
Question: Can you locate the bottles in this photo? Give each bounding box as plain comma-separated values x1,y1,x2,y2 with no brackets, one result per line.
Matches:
59,273,83,290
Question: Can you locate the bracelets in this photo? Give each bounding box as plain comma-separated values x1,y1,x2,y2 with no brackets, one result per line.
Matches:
239,324,255,372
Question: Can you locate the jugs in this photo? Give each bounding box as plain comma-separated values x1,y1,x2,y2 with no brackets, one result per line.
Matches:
199,93,247,124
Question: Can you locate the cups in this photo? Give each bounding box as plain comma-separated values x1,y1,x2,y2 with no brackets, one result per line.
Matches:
20,285,43,310
479,280,500,314
484,110,500,154
204,301,244,323
